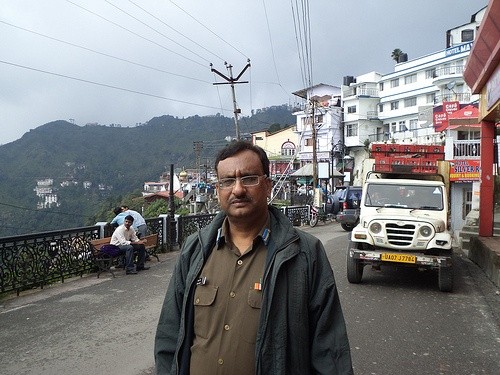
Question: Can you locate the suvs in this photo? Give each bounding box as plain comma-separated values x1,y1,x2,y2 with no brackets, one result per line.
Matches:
326,185,362,231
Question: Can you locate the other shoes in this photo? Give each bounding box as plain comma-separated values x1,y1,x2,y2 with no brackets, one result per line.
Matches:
126,270,138,274
115,264,134,268
145,256,151,261
136,266,150,271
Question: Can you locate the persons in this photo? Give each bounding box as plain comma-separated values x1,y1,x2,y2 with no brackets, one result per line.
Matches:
153,141,354,375
110,206,151,275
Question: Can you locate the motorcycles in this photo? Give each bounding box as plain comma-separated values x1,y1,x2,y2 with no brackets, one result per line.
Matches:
306,202,330,227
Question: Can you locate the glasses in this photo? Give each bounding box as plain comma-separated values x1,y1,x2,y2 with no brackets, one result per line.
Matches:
218,174,267,189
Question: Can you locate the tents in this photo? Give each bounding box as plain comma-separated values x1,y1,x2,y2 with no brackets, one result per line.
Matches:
288,164,345,204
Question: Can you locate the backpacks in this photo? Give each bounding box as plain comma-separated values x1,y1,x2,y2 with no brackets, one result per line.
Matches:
100,244,121,258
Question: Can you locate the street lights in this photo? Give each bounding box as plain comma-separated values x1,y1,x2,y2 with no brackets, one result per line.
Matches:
330,140,346,193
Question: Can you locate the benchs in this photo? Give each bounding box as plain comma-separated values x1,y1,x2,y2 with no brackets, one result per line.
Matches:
140,233,162,264
87,237,127,278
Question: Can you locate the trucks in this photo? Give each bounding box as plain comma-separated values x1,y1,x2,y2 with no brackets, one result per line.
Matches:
346,143,455,293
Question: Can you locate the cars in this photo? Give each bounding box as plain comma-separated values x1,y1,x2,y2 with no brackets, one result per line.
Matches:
297,185,314,196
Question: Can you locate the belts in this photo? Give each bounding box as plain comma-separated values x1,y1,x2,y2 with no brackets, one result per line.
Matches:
137,224,145,228
